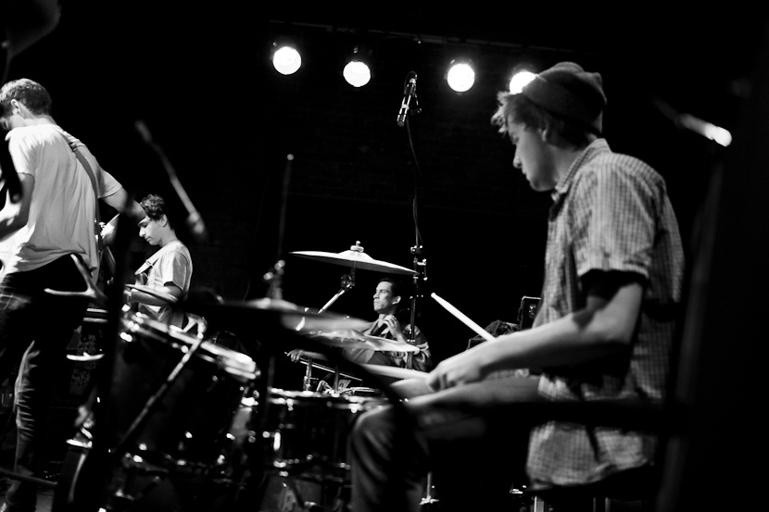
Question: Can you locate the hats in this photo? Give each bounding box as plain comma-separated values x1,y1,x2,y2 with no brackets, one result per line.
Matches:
522,62,606,136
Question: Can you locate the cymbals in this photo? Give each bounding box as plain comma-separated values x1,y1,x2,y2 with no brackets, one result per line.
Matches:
220,297,345,320
282,316,421,354
290,247,419,278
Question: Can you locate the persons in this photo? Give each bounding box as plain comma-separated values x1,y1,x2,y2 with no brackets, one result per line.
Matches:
345,61,688,511
347,279,432,373
1,78,146,512
124,195,192,418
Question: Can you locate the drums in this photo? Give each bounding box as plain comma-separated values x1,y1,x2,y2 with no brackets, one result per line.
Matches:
98,453,257,512
107,318,258,472
324,384,394,472
258,389,326,471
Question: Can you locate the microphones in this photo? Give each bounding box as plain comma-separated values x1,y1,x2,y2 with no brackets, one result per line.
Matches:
129,119,222,268
395,70,420,128
